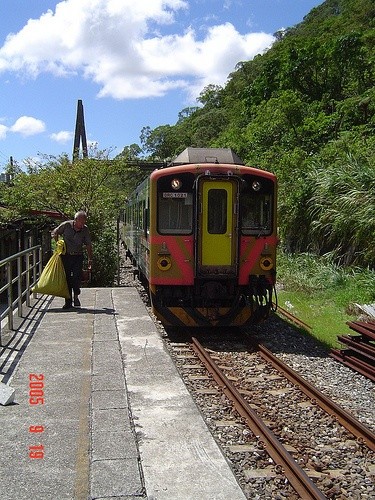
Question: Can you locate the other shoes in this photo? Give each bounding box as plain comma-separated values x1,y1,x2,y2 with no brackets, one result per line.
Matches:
74,300,81,306
62,303,72,308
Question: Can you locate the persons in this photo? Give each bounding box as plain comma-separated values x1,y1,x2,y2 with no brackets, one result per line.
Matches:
53,212,94,310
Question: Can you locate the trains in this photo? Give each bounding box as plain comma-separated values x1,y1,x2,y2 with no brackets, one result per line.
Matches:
118,146,280,331
